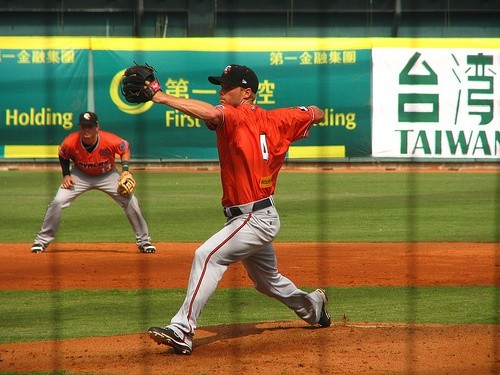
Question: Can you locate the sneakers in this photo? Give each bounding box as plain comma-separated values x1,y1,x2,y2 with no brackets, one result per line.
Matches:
148,327,192,355
31,244,44,253
140,244,155,253
315,288,331,327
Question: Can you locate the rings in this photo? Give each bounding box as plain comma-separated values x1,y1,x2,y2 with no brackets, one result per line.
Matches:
64,186,68,189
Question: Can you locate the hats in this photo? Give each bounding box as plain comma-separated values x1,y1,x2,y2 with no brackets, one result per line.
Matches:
208,65,258,93
77,112,99,126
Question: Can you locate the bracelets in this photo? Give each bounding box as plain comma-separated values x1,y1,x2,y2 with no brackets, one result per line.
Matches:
122,160,129,173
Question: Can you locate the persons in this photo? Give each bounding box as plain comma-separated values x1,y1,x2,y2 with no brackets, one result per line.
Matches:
30,110,158,254
144,63,332,355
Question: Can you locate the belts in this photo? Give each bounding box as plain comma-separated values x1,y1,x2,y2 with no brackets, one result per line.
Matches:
223,197,274,217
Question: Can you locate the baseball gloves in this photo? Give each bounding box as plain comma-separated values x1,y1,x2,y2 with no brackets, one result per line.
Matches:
119,60,162,104
117,171,136,196
